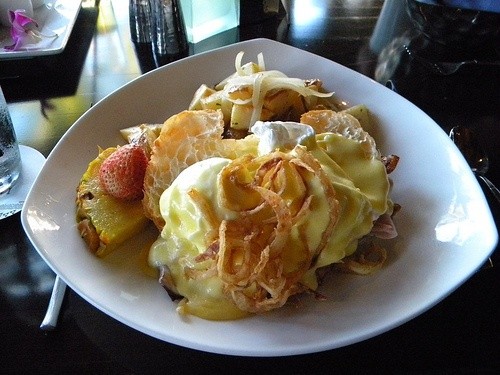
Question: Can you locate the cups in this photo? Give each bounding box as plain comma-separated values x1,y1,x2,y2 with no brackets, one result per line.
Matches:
0,85,22,194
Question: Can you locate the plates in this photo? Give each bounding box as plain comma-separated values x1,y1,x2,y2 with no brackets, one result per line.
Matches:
20,38,500,357
0,0,82,61
0,143,47,221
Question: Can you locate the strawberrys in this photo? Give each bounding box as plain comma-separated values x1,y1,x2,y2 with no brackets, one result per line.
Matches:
99,144,149,201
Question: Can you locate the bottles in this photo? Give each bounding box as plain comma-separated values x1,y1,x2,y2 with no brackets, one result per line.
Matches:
151,0,190,53
129,0,151,44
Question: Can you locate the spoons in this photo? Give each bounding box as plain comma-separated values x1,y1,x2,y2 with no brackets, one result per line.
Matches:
449,124,500,210
